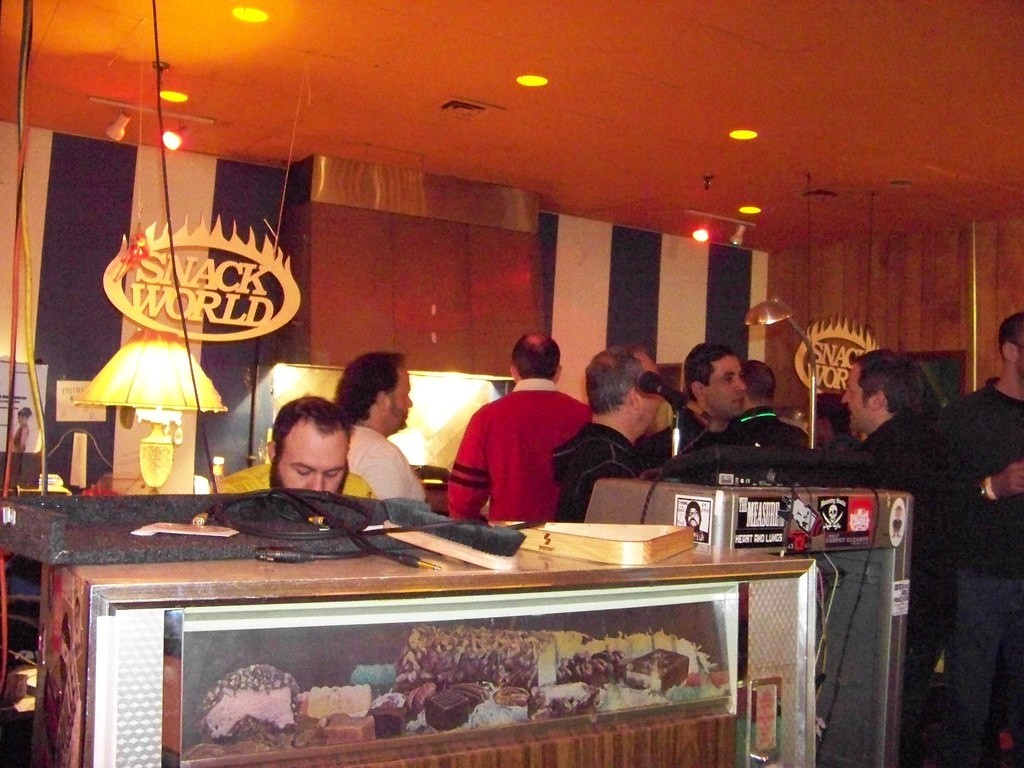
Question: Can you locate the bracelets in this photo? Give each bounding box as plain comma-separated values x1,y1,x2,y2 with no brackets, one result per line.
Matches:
984,475,996,501
977,483,985,495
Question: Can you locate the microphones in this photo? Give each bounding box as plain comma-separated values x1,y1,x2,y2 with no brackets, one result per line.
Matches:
637,369,687,411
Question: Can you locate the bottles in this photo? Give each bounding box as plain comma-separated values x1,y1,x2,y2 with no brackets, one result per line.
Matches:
211,456,226,483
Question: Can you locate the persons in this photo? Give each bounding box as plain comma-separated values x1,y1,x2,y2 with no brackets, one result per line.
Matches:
448,334,595,523
553,312,1024,768
212,353,427,502
12,408,33,451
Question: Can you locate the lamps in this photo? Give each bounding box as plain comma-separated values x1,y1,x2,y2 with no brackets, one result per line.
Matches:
105,108,132,142
744,295,815,450
75,331,228,494
728,224,746,247
163,126,192,150
